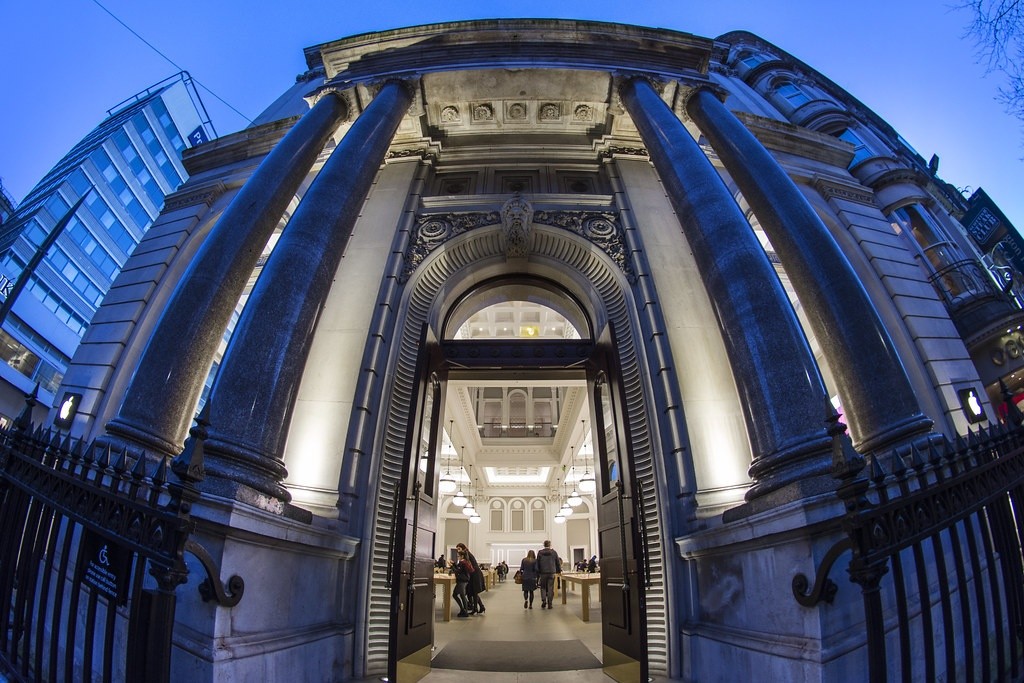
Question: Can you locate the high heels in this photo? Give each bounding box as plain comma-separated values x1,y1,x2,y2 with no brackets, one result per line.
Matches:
478,605,485,613
468,606,478,615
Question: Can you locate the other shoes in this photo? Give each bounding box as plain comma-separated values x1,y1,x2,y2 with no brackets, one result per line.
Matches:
541,603,546,608
524,601,528,608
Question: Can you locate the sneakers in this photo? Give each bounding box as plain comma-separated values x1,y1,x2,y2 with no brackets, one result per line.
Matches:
457,608,468,617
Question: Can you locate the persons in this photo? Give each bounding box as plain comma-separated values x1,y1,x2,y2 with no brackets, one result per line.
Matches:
588,556,597,573
495,561,509,581
520,550,538,609
456,543,486,616
575,559,588,571
434,554,446,573
537,540,562,609
448,551,473,617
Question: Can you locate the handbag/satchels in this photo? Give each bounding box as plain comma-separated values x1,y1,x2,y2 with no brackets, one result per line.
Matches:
513,568,524,584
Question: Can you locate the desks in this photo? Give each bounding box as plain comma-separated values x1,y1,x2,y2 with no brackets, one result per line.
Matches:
435,567,499,621
552,569,601,622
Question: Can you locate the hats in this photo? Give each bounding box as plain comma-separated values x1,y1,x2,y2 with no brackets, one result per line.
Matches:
594,556,597,558
584,559,586,561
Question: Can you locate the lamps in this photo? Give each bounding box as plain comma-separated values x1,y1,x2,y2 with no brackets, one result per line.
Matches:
452,446,468,506
470,480,482,523
438,418,456,493
567,445,583,506
462,464,476,515
554,478,566,523
579,419,596,492
560,464,573,516
